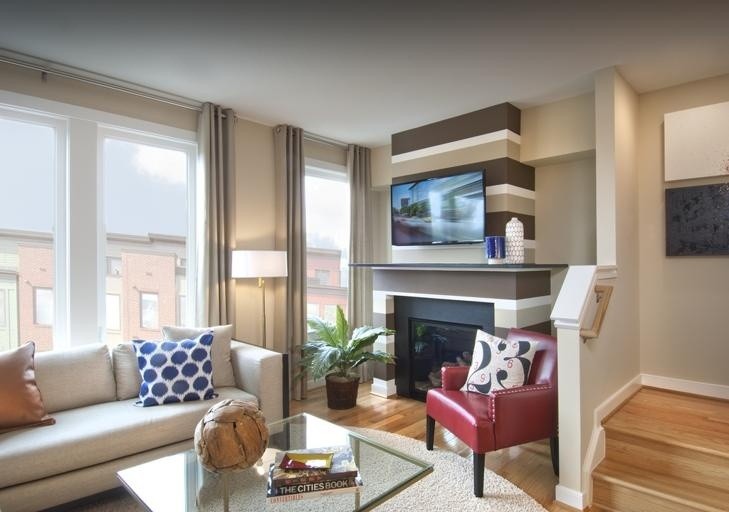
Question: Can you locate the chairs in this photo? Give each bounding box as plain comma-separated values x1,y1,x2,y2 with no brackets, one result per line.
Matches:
426,328,558,497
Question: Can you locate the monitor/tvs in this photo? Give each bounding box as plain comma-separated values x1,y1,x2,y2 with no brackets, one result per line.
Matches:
389,168,486,246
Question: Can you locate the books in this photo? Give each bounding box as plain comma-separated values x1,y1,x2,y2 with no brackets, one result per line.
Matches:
271,445,360,484
265,479,363,498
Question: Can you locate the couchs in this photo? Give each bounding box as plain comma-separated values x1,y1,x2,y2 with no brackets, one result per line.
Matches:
0,339,288,512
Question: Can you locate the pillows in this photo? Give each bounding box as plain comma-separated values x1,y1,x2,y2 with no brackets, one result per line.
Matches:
0,341,55,434
459,328,539,396
133,330,218,407
161,325,236,387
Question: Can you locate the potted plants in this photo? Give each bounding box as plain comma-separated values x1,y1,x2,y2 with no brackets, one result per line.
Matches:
291,305,400,410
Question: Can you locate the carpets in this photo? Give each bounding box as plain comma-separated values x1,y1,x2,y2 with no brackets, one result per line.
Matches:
196,427,550,512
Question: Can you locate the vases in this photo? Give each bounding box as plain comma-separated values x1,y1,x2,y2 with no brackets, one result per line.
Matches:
506,218,524,264
486,237,506,265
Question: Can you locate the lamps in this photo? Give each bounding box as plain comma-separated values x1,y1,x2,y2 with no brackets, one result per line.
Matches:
232,250,288,347
